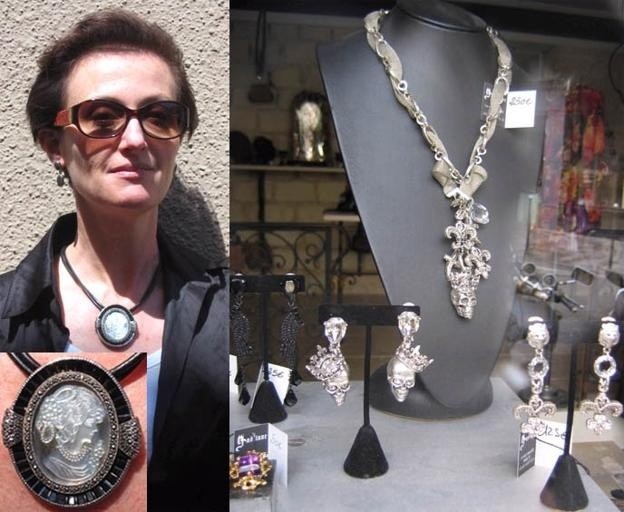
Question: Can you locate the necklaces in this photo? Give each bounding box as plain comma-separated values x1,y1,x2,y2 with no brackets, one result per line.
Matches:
365,8,512,321
1,351,148,509
60,243,162,350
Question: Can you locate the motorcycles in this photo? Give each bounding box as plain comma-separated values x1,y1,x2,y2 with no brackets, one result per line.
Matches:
496,248,623,405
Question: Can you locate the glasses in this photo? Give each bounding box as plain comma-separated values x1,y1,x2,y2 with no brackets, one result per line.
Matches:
52,99,191,139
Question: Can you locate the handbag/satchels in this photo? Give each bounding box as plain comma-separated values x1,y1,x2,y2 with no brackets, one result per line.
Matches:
250,87,273,103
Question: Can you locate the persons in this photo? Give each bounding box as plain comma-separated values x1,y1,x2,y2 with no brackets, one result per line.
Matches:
0,10,229,512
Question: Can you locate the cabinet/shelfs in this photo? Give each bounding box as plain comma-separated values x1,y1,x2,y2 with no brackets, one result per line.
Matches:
230,162,362,223
